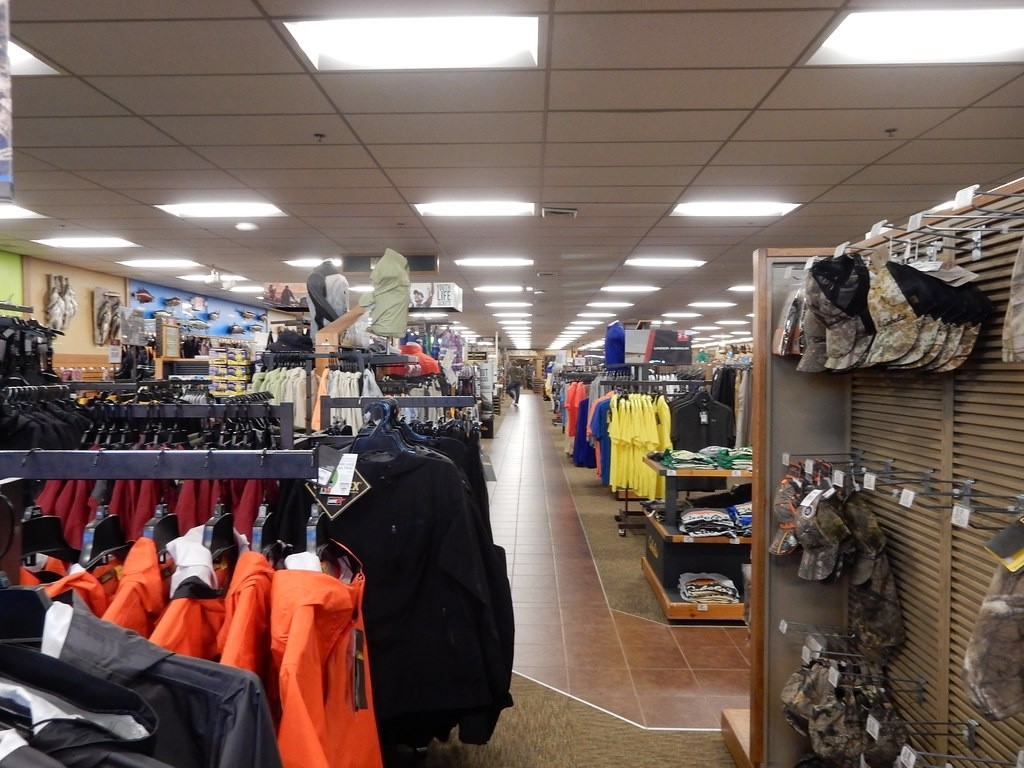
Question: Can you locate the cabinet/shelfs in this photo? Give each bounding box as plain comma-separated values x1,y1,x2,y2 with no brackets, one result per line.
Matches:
639,457,753,620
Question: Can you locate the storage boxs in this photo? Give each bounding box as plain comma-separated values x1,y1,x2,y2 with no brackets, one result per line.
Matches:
645,517,738,588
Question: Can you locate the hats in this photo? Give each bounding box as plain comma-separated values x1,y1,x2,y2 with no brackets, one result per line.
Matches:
767,475,886,584
795,251,988,374
781,662,906,763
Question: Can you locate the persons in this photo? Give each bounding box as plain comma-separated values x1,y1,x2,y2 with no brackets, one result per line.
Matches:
267,285,276,299
280,286,296,305
506,359,523,406
413,283,434,307
545,361,562,411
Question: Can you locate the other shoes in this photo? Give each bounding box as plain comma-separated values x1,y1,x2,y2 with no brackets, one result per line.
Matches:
514,403,517,406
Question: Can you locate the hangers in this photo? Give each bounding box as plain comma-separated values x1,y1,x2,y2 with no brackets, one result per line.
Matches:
558,361,753,402
0,302,481,646
